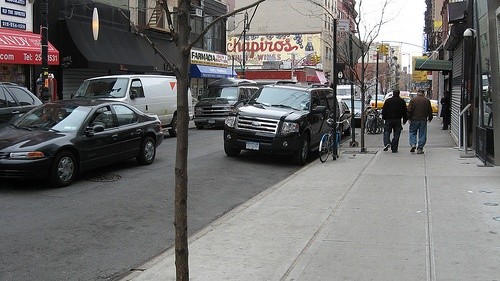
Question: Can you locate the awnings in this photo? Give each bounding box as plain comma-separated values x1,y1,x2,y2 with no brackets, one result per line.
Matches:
415,58,453,70
59,20,154,72
0,28,59,65
144,30,177,74
190,64,238,79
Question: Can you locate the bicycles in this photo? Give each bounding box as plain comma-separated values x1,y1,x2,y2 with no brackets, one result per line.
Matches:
318,118,350,163
365,107,385,134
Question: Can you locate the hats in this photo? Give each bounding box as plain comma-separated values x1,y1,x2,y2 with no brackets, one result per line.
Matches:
418,90,424,94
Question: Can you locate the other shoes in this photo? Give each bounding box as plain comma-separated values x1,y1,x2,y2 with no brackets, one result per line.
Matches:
410,143,416,152
383,144,391,151
417,148,424,154
392,149,398,153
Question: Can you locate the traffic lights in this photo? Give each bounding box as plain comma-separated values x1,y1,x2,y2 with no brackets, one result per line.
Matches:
377,44,383,53
316,55,320,64
311,55,315,64
383,42,389,56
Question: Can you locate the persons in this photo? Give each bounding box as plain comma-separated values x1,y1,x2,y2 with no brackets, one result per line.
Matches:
382,89,408,152
440,91,450,130
408,90,433,154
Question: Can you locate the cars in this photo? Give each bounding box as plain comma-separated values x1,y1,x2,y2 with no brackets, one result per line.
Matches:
0,97,165,188
430,99,438,114
400,96,412,113
335,84,385,128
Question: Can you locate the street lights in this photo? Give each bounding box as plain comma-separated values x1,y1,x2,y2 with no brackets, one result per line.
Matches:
243,12,251,79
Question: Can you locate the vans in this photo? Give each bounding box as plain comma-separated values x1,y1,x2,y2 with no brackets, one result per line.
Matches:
71,74,193,137
193,77,261,130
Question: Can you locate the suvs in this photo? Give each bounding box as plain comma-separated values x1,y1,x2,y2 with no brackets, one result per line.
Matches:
0,80,44,129
223,82,342,167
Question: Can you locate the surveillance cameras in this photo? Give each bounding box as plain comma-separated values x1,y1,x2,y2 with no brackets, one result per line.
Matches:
463,29,472,40
